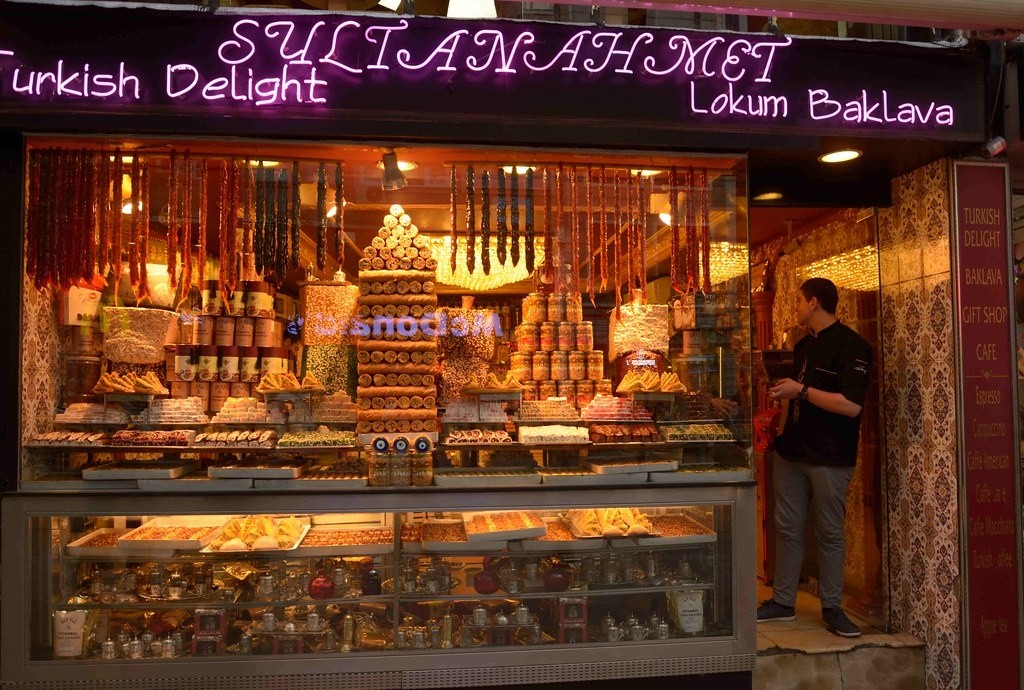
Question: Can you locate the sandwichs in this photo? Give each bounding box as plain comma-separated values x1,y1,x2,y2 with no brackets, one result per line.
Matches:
210,514,305,551
566,508,653,536
256,371,319,389
461,372,524,389
615,369,687,393
91,372,169,395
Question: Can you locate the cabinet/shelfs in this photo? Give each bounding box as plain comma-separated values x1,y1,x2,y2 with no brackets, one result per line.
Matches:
0,416,756,690
672,291,736,332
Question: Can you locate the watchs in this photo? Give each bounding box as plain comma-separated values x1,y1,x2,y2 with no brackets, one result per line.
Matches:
799,385,809,399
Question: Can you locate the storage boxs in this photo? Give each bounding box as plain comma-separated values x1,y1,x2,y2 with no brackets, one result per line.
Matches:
60,286,102,326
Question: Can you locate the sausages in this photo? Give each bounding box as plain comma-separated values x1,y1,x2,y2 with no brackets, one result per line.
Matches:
28,145,345,314
450,162,710,322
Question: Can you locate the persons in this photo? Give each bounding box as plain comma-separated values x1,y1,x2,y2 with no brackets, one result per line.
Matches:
669,291,745,437
757,278,868,638
282,325,304,355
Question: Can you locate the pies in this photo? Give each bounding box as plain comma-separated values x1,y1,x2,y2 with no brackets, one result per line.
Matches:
443,428,512,444
29,431,115,446
194,428,277,448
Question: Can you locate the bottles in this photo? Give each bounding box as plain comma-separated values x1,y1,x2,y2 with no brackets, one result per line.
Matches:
666,561,704,636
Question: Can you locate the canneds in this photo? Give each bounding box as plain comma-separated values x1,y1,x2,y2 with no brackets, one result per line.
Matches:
501,292,613,413
165,280,288,411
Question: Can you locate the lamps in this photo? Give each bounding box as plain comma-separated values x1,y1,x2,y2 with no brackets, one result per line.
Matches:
698,240,748,284
590,4,606,26
659,192,687,227
380,150,407,190
324,183,346,216
400,0,414,15
945,29,976,47
710,174,739,211
200,0,219,14
415,228,557,291
762,16,781,34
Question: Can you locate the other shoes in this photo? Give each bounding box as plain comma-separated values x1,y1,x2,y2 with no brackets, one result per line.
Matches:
822,609,861,636
757,599,795,622
